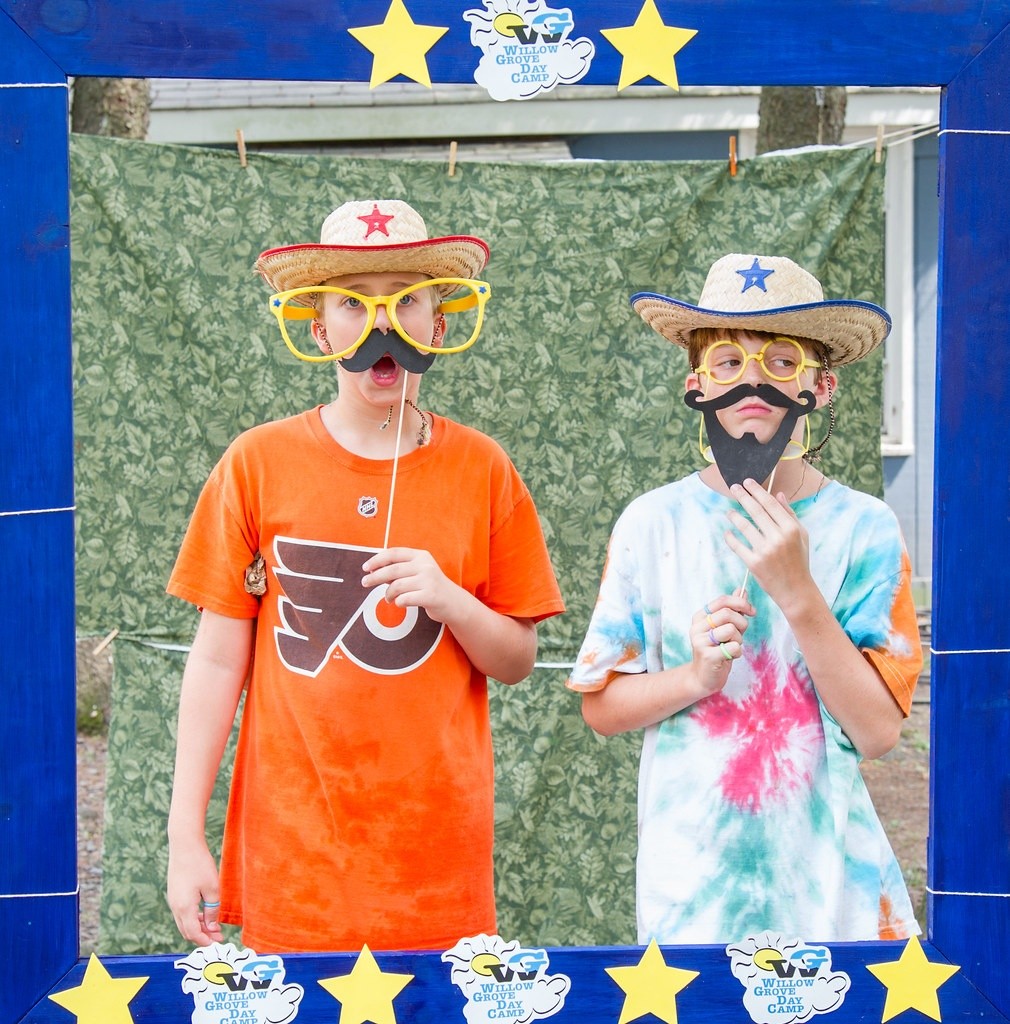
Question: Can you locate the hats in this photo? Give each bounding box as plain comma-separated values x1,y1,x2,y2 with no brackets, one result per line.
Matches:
631,252,892,367
253,199,492,306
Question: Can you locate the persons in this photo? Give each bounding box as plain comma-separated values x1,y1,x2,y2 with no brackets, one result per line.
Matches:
565,254,925,946
167,200,564,954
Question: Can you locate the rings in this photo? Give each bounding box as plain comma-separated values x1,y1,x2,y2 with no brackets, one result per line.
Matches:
709,629,720,645
707,615,716,628
204,901,219,907
719,643,733,660
704,605,711,614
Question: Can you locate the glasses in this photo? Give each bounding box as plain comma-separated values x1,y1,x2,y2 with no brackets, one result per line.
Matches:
696,338,826,385
269,278,491,362
